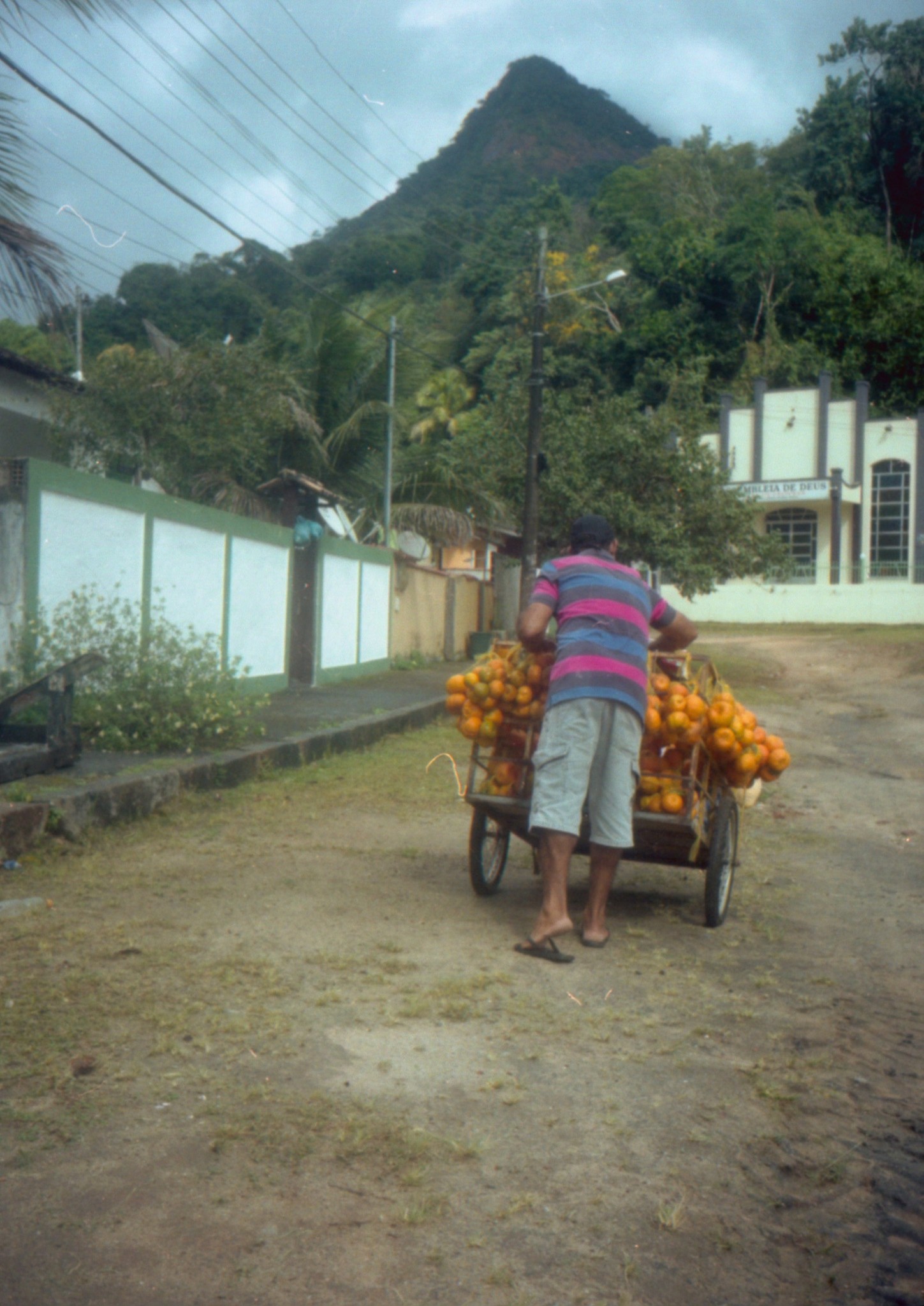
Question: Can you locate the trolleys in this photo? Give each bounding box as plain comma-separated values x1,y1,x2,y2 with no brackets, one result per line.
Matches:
460,650,743,928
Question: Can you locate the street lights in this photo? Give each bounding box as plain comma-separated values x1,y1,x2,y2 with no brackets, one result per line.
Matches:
75,295,128,381
518,267,631,617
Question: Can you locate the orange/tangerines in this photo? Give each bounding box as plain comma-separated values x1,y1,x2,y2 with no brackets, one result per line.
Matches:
444,649,790,816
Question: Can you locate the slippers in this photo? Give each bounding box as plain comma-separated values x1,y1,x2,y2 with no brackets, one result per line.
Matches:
514,936,575,963
581,925,610,948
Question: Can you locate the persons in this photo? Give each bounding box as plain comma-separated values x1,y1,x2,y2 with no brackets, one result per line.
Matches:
509,513,698,964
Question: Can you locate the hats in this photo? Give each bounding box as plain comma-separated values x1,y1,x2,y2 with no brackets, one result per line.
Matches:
572,514,612,544
